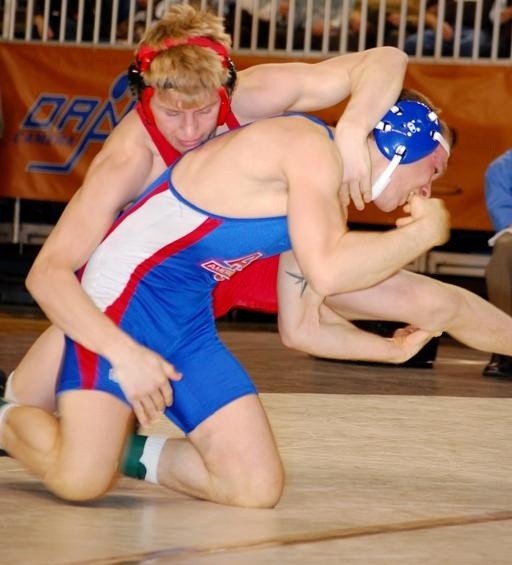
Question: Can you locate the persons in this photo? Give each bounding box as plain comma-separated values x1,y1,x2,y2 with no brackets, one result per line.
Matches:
1,0,511,62
482,147,512,381
0,85,457,514
1,0,512,462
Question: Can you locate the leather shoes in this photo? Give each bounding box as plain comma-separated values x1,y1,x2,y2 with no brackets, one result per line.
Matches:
482,354,511,376
